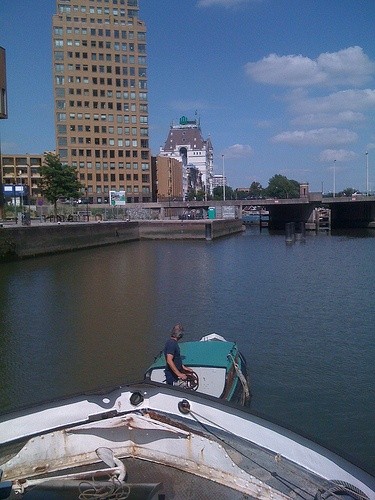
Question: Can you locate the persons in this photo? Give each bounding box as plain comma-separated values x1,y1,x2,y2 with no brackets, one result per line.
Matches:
164,324,191,385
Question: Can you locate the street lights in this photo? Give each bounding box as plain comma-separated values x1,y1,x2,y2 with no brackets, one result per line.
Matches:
333,160,337,198
221,154,226,201
365,152,369,195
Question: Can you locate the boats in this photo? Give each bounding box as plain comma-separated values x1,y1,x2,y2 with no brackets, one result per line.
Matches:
1,376,375,500
144,332,253,408
